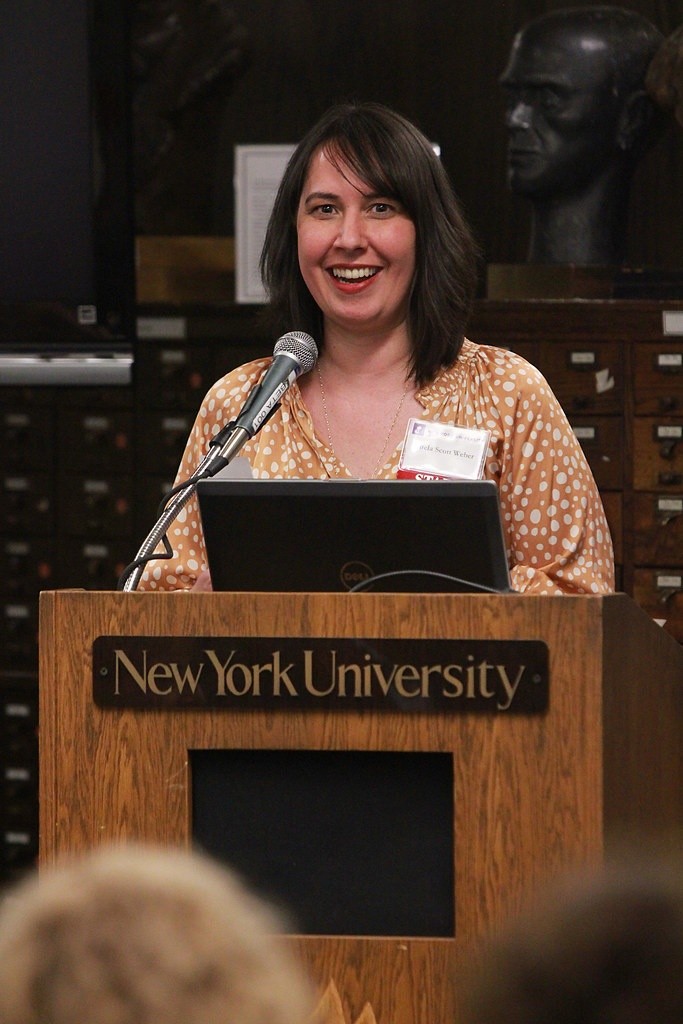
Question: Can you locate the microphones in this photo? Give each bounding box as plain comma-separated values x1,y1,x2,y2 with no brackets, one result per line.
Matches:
206,331,318,476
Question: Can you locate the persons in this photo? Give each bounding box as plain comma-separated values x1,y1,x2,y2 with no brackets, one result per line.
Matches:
0,843,309,1024
135,100,615,595
498,5,661,269
462,872,683,1024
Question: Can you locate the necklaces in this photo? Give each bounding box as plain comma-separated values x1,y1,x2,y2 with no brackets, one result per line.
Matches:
317,359,409,479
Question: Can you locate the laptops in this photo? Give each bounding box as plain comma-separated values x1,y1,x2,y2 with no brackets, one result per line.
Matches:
197,478,511,596
